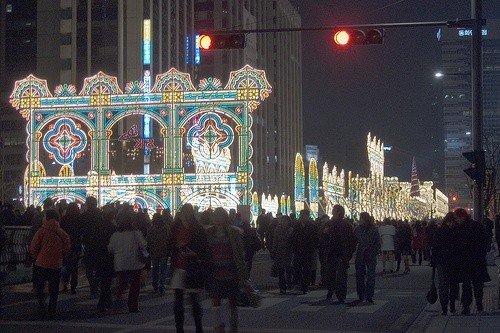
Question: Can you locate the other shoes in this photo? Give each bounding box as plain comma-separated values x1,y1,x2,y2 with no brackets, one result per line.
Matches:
25,267,490,321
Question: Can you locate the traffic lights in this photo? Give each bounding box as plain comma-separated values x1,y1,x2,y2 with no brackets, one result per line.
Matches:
462,150,486,184
198,34,246,50
333,28,384,46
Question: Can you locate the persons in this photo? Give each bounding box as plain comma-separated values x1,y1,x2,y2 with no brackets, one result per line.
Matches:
0,197,491,333
483,215,494,251
495,214,500,258
